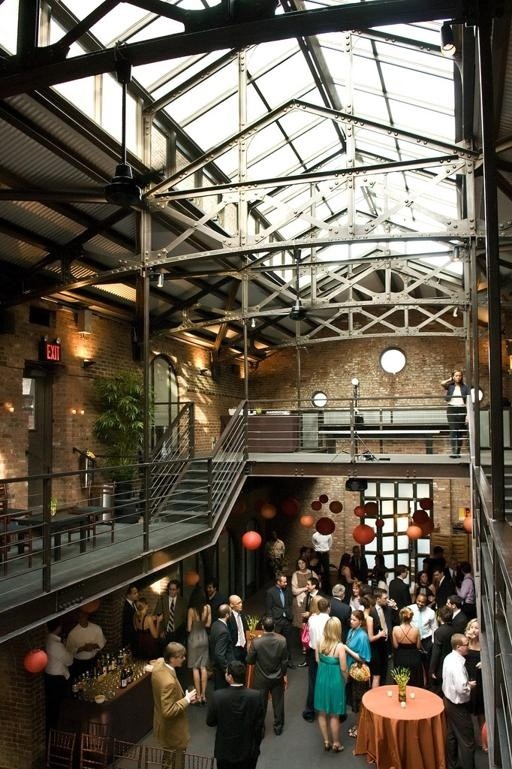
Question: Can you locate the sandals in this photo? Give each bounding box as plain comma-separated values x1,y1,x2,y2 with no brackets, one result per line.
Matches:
302,646,306,655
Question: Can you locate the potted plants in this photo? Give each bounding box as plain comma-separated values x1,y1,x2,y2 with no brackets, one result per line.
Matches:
90,370,158,524
244,614,261,640
389,664,412,703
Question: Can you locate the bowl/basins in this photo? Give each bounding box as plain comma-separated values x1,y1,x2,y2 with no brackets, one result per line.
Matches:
94,695,105,704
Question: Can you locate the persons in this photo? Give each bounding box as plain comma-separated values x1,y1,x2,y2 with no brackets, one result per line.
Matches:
441,370,471,459
151,642,202,769
206,662,264,769
389,558,477,694
266,548,402,755
245,615,288,736
121,576,249,706
66,610,106,677
43,623,74,744
429,596,485,768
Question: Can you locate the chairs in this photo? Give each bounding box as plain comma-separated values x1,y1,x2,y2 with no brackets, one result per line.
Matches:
46,730,215,769
0,481,32,577
68,480,115,545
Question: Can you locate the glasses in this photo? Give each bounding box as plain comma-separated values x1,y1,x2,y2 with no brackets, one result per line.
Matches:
459,643,469,646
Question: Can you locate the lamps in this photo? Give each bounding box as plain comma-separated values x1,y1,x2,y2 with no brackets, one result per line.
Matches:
0,402,14,412
454,305,466,317
299,346,309,355
346,478,368,490
200,367,207,374
351,377,359,453
251,318,256,328
453,245,467,260
79,355,97,369
146,272,164,288
440,20,464,57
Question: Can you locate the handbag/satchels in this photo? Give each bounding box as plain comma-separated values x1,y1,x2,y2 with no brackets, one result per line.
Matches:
349,661,371,682
301,624,310,650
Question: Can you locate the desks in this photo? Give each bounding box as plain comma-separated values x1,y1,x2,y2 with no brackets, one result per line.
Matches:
220,412,300,453
12,505,110,561
240,630,284,700
480,410,510,448
351,685,448,769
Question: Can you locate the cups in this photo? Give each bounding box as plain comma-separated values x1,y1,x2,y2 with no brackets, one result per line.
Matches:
387,600,395,606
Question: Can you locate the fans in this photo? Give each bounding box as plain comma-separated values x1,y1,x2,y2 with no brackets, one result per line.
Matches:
258,249,337,320
12,60,180,204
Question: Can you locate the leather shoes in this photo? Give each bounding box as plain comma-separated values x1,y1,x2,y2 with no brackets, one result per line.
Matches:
302,711,314,722
339,714,348,724
297,661,308,667
287,662,296,669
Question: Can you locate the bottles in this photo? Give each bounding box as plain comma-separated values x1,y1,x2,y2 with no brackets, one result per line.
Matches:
112,653,116,672
106,654,112,673
96,659,104,682
85,670,92,688
72,679,79,700
123,648,128,664
118,650,124,667
120,668,128,688
77,676,84,696
82,673,88,695
101,655,108,673
126,668,132,683
129,666,134,682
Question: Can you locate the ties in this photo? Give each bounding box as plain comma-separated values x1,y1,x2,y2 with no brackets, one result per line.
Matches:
356,557,359,570
132,602,135,609
418,610,424,639
379,610,386,631
308,596,312,608
167,599,175,632
236,615,246,648
452,570,455,581
280,589,286,617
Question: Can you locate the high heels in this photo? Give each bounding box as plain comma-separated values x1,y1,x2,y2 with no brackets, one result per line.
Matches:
332,745,344,753
348,725,358,732
190,697,207,707
323,742,333,751
348,730,357,738
